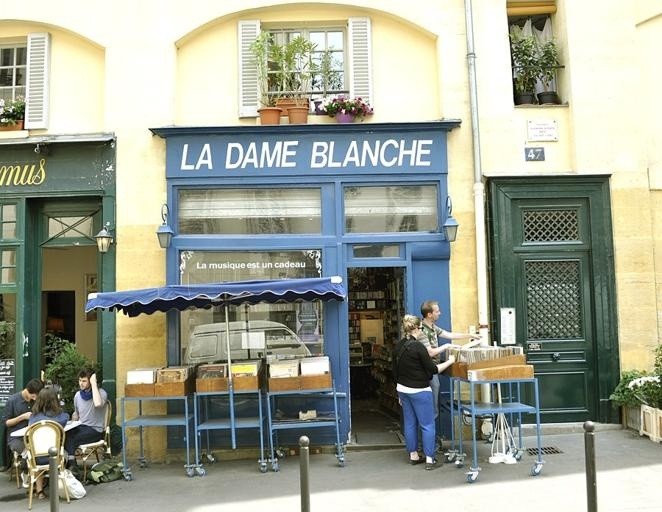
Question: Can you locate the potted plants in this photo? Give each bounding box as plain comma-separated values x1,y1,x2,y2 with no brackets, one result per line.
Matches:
609,344,662,444
315,46,342,115
249,23,315,126
510,23,561,106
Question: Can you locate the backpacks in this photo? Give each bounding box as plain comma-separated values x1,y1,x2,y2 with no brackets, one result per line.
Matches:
86,458,125,484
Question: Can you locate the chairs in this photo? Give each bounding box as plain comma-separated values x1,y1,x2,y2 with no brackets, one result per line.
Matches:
10,400,112,510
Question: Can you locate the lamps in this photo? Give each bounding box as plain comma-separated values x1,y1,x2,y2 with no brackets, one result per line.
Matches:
94,226,114,253
155,203,174,248
444,195,458,242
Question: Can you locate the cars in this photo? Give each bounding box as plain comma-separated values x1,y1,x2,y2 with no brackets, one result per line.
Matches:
298,325,319,342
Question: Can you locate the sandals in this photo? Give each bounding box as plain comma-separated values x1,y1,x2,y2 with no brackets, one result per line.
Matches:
34,490,46,499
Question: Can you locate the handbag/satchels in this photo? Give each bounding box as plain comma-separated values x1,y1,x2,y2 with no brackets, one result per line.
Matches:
58,468,87,499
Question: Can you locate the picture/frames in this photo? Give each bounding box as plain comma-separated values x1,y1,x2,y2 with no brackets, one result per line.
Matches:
83,272,99,322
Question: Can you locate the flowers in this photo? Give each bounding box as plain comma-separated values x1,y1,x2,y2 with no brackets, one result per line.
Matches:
0,92,26,128
318,92,374,122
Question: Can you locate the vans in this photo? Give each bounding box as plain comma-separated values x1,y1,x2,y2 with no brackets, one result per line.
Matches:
183,320,312,365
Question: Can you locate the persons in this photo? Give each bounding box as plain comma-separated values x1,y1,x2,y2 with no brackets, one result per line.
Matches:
418,300,480,450
3,378,45,488
66,368,108,470
28,386,69,498
392,314,456,471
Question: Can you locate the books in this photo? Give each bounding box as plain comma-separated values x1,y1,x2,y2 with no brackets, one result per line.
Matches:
124,356,330,383
443,344,523,365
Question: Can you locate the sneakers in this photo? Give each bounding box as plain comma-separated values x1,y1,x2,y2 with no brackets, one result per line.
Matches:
409,455,426,464
425,458,442,469
21,471,30,488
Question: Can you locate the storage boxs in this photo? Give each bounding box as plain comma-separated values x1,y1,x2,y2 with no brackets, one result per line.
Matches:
125,365,334,397
443,354,534,380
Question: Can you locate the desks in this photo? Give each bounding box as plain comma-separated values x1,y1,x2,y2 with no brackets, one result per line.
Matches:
437,373,543,482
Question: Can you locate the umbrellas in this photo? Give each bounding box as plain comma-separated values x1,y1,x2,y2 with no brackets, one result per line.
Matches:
84,275,347,454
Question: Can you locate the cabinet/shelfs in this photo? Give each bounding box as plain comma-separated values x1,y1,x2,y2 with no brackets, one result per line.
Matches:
121,383,347,482
348,267,403,424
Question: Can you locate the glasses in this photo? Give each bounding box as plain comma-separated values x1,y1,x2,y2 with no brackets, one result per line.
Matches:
418,327,424,331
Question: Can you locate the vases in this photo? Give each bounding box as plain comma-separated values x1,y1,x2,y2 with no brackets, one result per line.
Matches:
0,119,24,131
336,111,353,124
